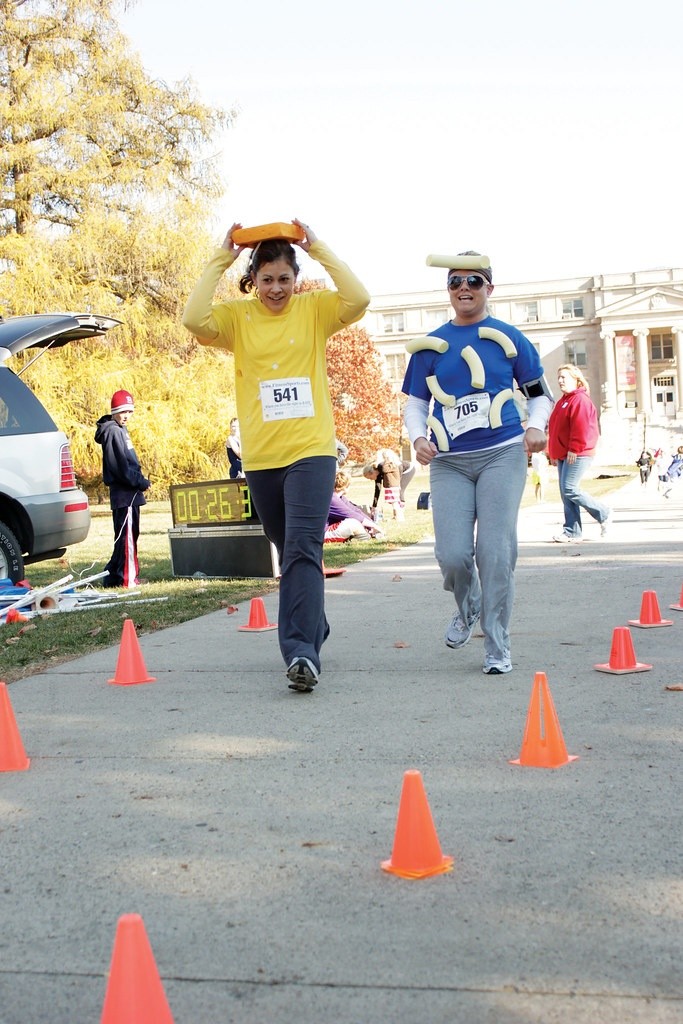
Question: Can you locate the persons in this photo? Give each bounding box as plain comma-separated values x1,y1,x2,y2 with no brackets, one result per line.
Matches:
636,445,683,498
323,438,416,545
402,249,553,673
226,417,244,479
94,390,150,588
181,217,372,691
547,363,614,543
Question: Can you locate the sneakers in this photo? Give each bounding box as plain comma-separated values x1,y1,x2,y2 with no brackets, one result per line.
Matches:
483,649,514,675
445,606,481,649
286,658,319,694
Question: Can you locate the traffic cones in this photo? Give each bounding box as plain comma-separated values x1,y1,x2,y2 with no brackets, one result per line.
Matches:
628,590,673,629
237,598,278,632
592,627,654,675
108,619,155,685
380,769,454,880
101,915,173,1024
0,682,31,771
670,585,683,611
508,672,579,768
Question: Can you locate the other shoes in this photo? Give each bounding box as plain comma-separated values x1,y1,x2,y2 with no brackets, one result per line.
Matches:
601,508,614,536
553,532,584,544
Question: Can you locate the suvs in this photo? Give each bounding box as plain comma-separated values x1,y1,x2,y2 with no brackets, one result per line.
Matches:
0,312,124,588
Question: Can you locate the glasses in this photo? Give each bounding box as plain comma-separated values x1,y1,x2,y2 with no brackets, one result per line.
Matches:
446,275,489,291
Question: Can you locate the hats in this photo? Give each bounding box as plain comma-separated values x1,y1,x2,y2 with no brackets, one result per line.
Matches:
111,390,135,413
448,250,493,282
